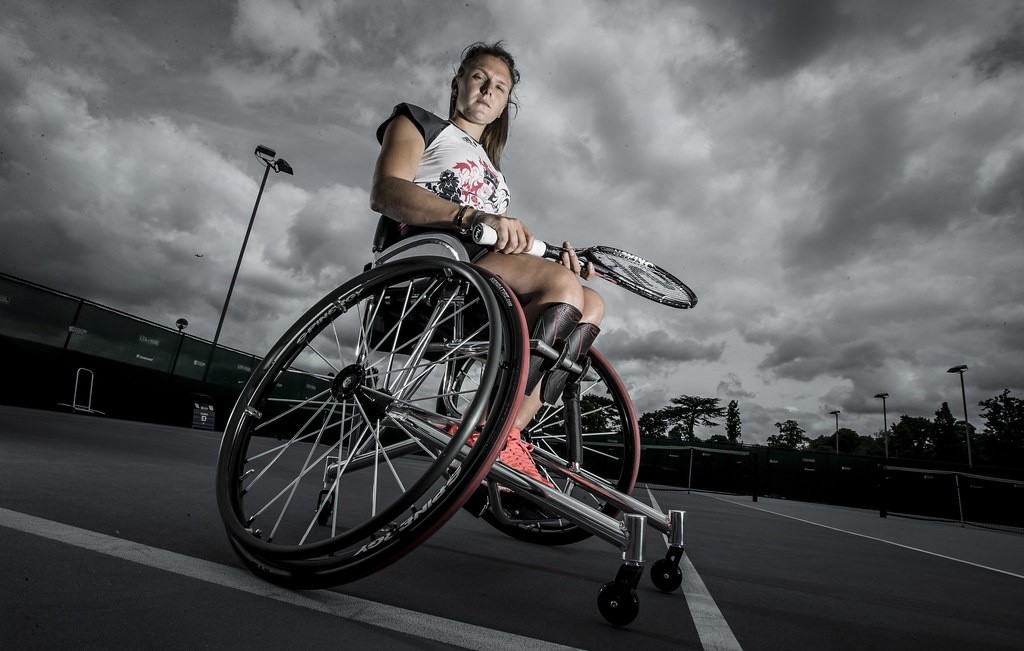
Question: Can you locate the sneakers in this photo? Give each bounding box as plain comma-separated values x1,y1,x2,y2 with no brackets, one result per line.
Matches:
445,423,555,493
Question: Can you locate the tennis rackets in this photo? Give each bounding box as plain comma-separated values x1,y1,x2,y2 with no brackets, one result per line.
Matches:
472,222,697,311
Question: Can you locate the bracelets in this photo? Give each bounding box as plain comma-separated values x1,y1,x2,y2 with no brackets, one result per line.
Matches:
452,204,485,233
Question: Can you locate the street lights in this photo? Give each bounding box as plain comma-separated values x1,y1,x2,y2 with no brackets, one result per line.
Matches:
946,364,974,466
874,393,889,459
828,410,841,454
198,143,296,401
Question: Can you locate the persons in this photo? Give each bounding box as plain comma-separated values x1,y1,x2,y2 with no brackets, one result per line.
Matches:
368,38,608,495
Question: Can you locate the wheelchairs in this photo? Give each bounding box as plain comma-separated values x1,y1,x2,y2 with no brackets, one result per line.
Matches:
214,216,687,625
